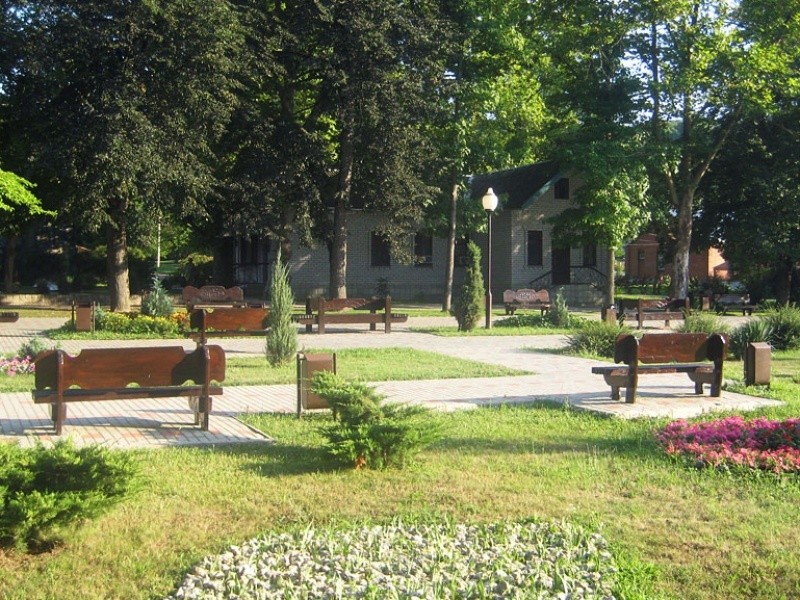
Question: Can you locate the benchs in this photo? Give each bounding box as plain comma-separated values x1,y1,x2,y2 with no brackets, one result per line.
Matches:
30,344,225,435
184,307,273,347
591,332,729,403
691,283,726,300
617,297,692,328
713,293,760,314
503,288,552,317
296,295,409,334
182,284,244,309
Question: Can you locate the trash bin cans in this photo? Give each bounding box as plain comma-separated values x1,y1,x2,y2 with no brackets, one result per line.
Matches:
744,341,771,388
702,295,710,311
297,354,337,421
142,289,150,304
73,300,95,333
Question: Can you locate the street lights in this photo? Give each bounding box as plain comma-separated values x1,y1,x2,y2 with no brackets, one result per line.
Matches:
482,188,499,329
